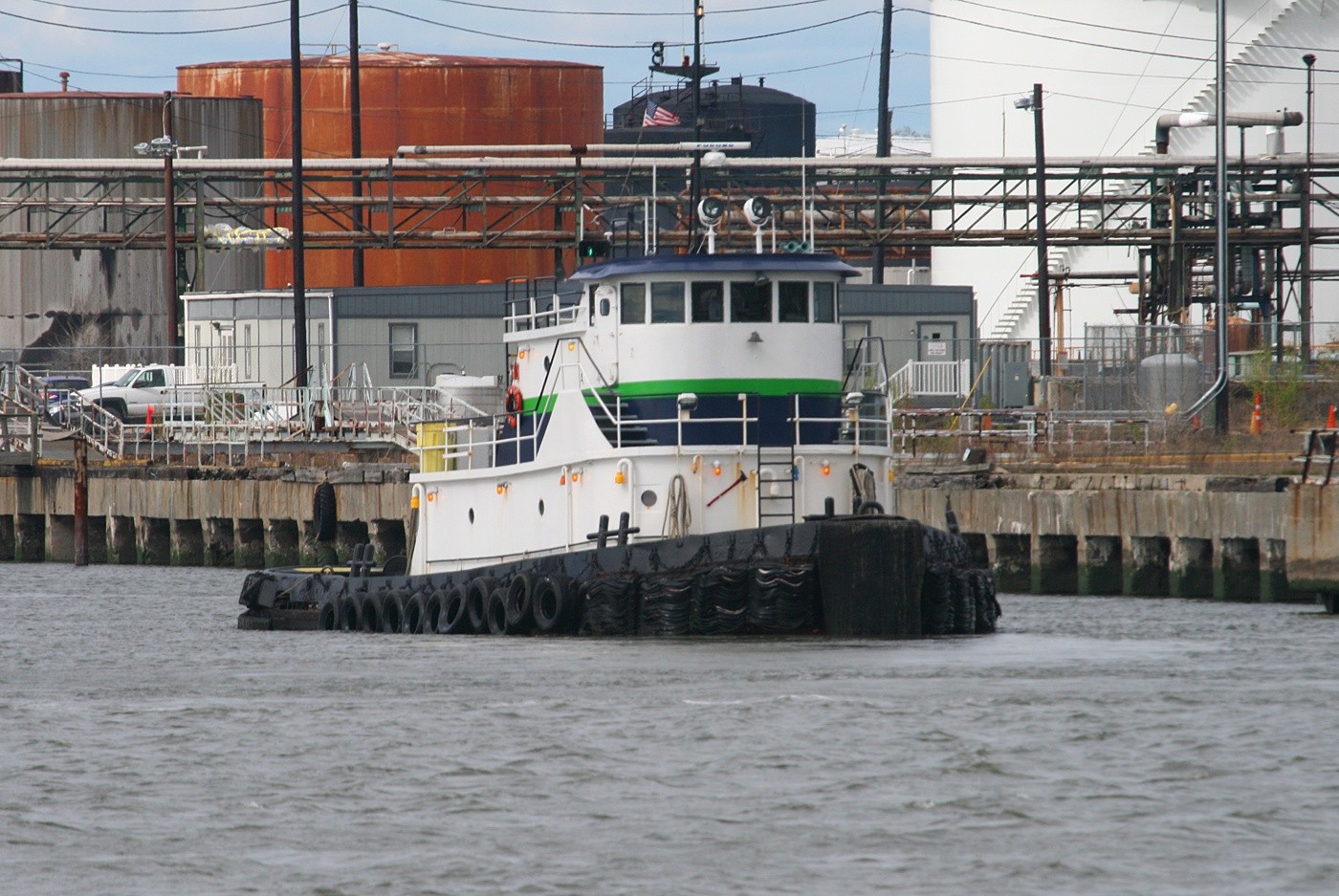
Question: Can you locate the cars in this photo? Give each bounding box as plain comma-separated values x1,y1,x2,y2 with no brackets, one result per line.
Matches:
32,375,91,427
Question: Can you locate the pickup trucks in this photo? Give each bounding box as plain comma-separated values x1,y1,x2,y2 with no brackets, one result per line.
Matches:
69,363,271,439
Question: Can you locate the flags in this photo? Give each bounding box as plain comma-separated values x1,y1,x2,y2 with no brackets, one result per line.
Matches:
642,97,680,129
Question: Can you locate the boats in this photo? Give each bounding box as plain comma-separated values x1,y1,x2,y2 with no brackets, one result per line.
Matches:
234,1,1005,644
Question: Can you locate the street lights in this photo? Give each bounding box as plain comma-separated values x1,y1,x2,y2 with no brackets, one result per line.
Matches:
1011,84,1052,374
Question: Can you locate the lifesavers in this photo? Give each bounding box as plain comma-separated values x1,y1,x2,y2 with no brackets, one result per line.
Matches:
316,570,574,635
505,387,523,425
